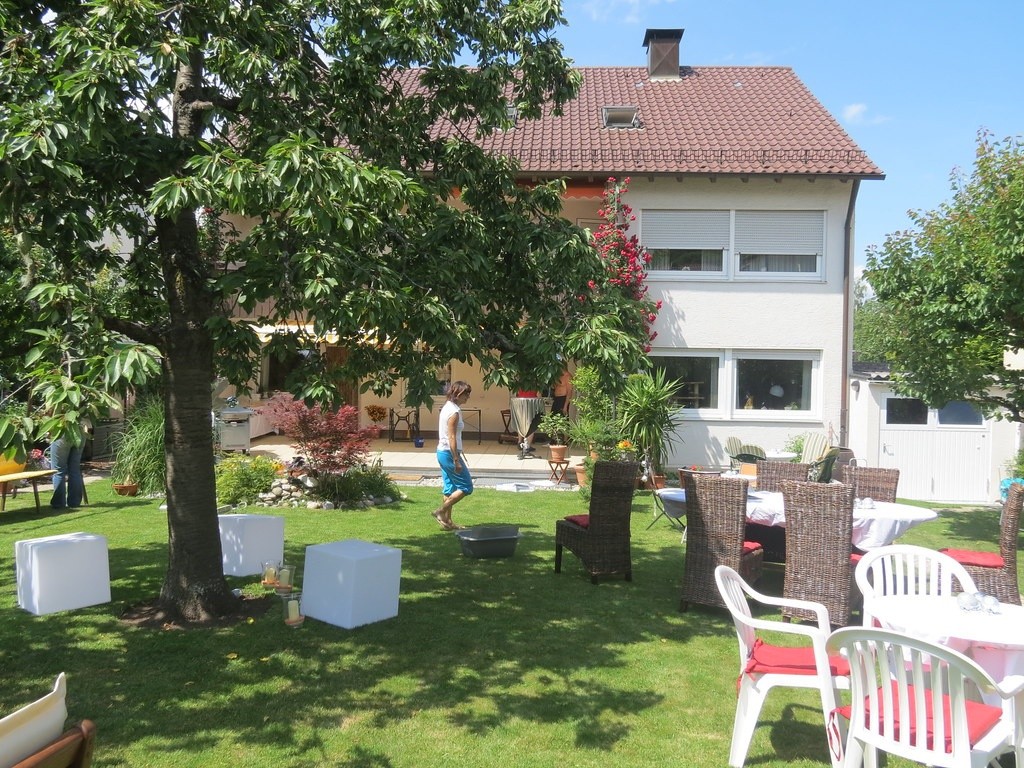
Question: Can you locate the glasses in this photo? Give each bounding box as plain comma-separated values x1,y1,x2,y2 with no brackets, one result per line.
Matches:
463,391,470,396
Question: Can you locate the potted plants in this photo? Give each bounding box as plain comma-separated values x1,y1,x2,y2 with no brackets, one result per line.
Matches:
620,369,688,487
566,421,608,487
365,405,388,439
538,411,572,462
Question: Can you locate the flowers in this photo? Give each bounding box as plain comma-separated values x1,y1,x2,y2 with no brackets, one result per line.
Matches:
618,439,634,452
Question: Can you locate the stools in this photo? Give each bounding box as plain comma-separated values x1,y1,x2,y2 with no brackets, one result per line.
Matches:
549,460,570,484
218,514,283,576
15,532,113,614
301,540,401,628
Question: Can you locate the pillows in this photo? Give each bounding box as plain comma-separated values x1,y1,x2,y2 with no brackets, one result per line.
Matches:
564,514,590,528
0,671,67,768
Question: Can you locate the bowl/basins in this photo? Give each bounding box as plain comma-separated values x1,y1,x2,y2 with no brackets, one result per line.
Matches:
455,526,522,558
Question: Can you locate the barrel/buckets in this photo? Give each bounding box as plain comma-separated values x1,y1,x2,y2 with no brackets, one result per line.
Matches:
414,437,424,447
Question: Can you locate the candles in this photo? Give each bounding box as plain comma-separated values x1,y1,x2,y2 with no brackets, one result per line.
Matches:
280,570,290,588
288,600,299,621
264,568,274,581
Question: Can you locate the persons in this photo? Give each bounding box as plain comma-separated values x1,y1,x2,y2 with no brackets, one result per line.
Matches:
430,381,474,529
47,359,85,510
516,364,572,453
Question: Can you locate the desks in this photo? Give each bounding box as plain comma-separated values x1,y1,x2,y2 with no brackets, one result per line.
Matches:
874,593,1024,709
0,468,57,514
245,413,279,439
654,487,938,552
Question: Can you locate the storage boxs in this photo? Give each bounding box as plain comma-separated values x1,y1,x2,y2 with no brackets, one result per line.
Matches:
458,529,519,559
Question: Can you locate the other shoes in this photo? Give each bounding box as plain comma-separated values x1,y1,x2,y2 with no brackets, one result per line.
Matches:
542,443,550,448
518,443,523,451
431,510,465,530
528,447,535,451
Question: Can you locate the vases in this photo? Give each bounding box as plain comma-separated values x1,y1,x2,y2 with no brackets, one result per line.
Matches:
621,450,633,463
114,483,139,496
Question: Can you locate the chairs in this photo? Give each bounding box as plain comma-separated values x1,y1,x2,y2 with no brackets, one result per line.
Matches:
554,463,637,583
13,718,96,768
647,460,1024,768
